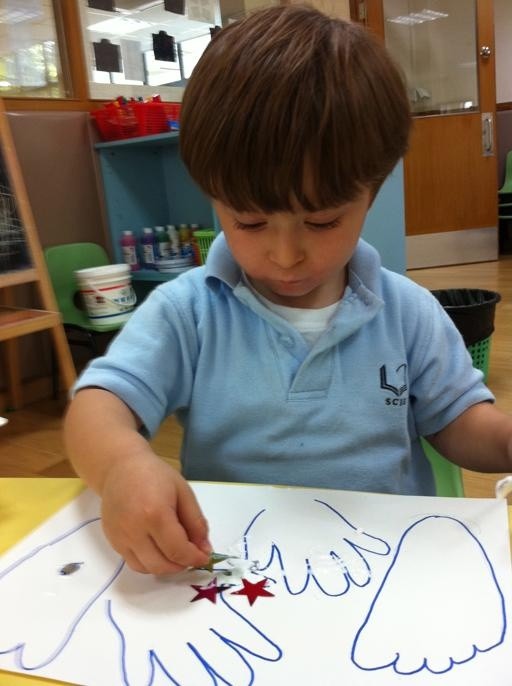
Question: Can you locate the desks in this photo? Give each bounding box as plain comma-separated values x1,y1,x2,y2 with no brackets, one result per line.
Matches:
0,476,512,686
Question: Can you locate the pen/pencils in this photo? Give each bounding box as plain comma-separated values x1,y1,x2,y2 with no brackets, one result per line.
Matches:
208,552,239,563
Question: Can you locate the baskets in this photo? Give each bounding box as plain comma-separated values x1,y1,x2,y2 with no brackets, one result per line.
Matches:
192,227,216,265
90,102,182,141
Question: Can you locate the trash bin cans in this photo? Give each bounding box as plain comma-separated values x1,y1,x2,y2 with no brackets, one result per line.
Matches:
430,288,501,385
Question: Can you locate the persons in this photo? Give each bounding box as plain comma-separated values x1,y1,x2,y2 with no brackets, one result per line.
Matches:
63,3,512,576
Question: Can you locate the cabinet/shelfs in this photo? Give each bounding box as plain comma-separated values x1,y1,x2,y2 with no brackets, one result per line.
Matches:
92,110,407,305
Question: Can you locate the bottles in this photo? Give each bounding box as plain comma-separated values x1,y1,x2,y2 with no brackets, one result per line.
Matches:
119,223,203,271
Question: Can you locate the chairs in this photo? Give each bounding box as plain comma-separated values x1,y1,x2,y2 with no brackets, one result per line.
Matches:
31,242,142,397
414,422,467,496
497,150,512,218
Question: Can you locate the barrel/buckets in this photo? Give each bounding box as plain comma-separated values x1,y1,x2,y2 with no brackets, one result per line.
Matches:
75,264,138,327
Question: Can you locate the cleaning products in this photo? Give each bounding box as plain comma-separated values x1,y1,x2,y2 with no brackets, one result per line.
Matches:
142,222,199,267
121,229,137,269
